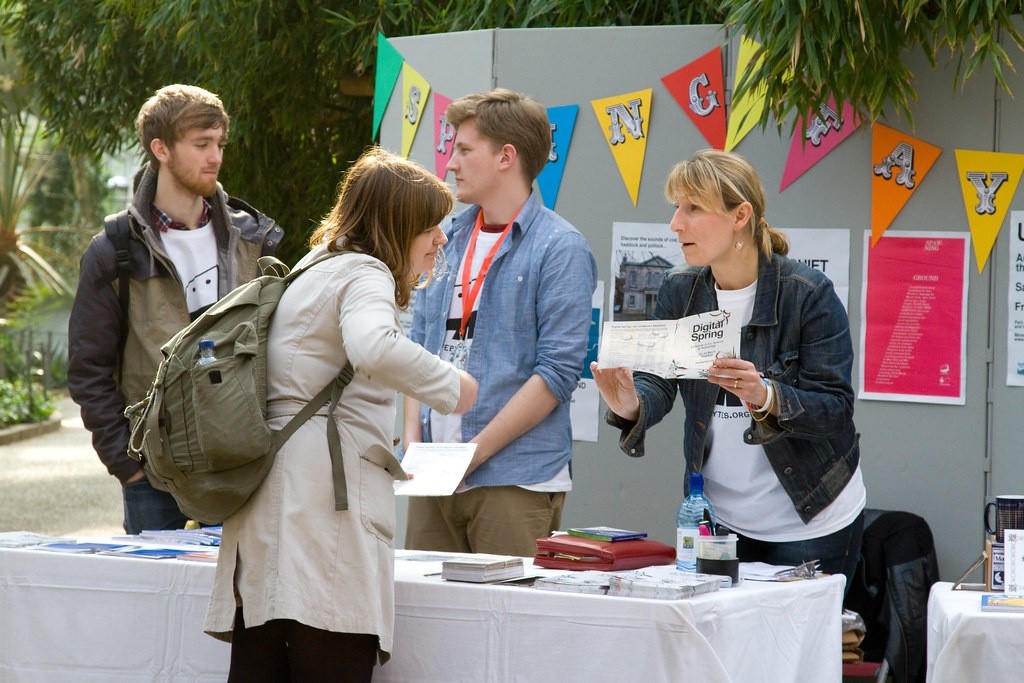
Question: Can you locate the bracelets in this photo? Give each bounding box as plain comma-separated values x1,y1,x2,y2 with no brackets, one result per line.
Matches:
746,378,775,422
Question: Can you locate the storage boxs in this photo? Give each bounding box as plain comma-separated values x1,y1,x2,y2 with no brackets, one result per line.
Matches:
984,540,1003,591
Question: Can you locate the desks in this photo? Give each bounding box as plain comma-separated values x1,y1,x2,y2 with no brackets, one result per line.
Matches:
0,530,847,683
925,581,1024,683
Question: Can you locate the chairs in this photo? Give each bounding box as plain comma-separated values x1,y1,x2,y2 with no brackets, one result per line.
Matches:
843,509,942,683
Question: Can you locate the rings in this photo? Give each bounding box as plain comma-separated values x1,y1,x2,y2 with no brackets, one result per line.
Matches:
733,380,739,388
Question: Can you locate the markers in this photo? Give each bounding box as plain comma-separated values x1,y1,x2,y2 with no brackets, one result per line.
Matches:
713,528,729,544
699,525,710,536
704,508,714,536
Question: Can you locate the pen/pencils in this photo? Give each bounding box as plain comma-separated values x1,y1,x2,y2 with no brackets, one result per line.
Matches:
712,372,764,379
424,573,442,576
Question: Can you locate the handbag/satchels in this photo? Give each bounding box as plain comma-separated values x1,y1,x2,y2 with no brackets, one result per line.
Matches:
533,535,676,571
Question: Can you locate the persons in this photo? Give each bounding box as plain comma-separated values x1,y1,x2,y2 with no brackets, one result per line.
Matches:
401,88,597,562
202,148,477,683
590,148,898,612
65,83,295,535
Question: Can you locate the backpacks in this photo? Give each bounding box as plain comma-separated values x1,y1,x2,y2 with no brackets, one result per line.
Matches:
124,250,354,526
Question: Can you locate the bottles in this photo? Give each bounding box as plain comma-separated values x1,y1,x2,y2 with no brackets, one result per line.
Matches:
676,472,716,573
195,340,218,368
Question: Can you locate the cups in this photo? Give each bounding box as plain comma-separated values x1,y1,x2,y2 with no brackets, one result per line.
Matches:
698,533,739,560
695,556,740,587
983,494,1024,543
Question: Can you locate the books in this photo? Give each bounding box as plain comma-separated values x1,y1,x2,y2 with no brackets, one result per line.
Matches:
109,527,222,547
